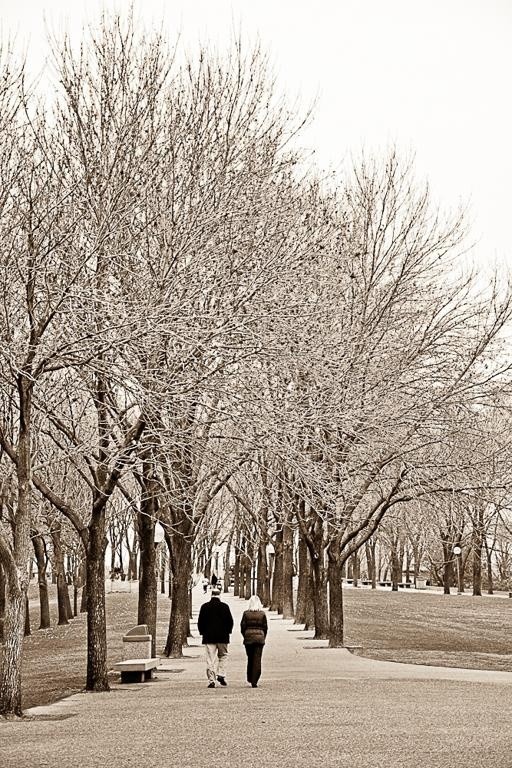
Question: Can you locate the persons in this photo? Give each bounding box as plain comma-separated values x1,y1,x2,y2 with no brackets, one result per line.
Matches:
198,589,233,688
202,574,222,594
240,596,268,688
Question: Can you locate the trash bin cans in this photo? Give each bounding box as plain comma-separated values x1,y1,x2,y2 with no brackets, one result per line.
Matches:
122,624,152,661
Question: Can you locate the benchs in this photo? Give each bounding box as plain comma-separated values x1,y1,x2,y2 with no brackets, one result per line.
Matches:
112,658,160,682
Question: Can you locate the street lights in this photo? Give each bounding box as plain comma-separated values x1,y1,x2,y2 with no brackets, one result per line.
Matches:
453,546,462,596
153,523,166,550
215,545,221,577
264,543,274,607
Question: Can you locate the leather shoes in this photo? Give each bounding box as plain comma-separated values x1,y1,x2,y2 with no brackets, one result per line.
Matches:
217,673,227,686
208,682,215,688
249,681,257,687
